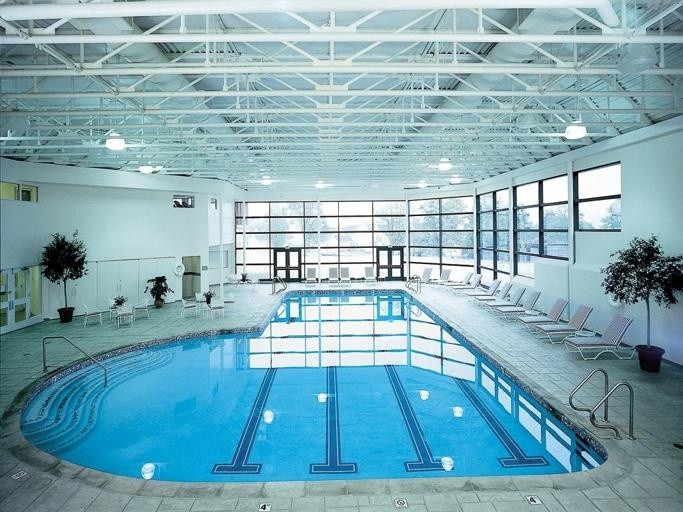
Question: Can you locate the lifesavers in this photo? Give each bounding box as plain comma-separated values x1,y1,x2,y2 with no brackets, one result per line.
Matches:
606,291,625,307
174,263,185,276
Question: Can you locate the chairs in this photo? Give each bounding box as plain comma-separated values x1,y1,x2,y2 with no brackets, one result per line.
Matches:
81,298,150,329
180,292,237,321
302,266,379,289
413,266,637,361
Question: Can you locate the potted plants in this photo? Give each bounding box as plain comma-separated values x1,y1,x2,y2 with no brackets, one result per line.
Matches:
35,229,89,323
202,289,217,304
144,274,175,308
240,272,248,282
113,296,128,306
598,230,683,374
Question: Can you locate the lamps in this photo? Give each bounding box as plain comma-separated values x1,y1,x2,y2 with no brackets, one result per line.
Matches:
617,29,659,74
